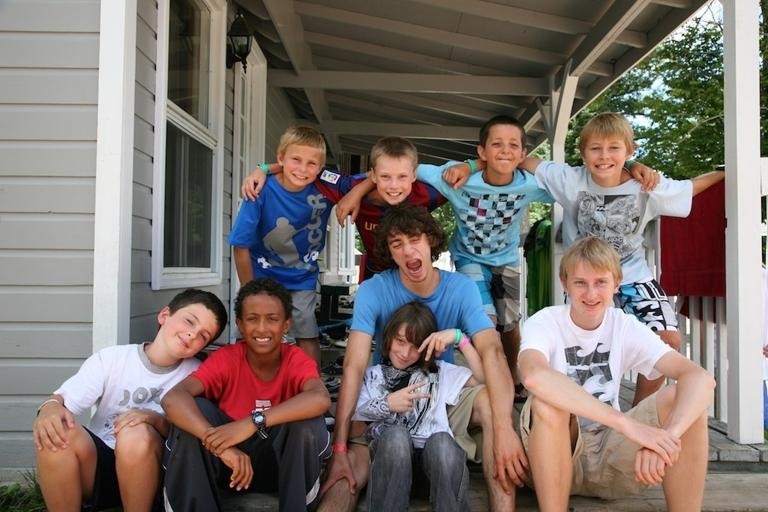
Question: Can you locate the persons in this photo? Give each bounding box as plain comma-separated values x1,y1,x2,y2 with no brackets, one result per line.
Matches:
242,135,447,283
227,123,364,431
509,232,716,512
162,277,334,511
443,111,724,408
314,201,531,511
348,296,485,512
32,287,227,511
335,115,656,403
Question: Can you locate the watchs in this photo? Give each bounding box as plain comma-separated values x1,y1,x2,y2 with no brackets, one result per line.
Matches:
251,410,269,440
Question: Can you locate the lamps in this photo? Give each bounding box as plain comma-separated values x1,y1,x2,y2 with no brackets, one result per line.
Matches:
226,0,254,73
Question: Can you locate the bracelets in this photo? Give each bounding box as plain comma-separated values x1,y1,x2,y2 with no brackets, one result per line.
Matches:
455,328,461,345
464,157,476,174
459,333,468,349
715,164,725,171
255,160,270,175
36,398,59,415
623,160,636,167
333,444,346,452
